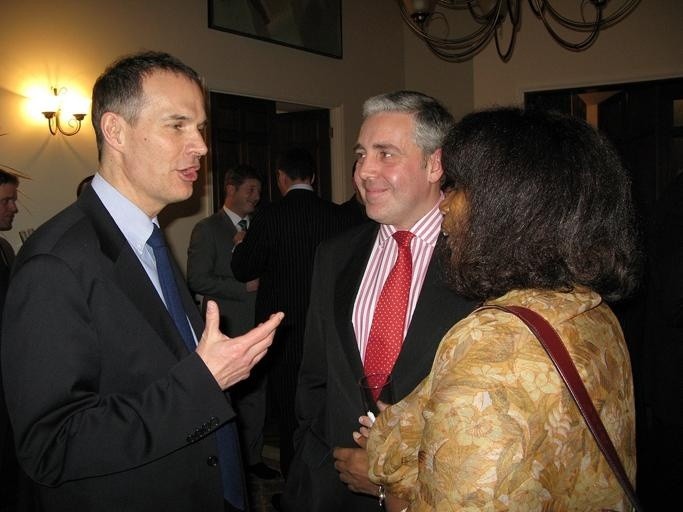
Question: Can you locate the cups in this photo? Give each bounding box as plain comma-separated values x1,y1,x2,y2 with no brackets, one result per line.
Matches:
358,373,395,418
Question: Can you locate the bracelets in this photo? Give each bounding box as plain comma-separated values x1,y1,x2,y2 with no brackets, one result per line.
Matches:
376,485,388,507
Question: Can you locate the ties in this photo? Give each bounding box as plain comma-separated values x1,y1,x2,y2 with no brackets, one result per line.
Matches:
238,218,248,229
363,230,418,401
147,222,244,510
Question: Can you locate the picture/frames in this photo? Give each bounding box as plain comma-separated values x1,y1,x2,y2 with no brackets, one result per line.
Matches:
207,0,343,60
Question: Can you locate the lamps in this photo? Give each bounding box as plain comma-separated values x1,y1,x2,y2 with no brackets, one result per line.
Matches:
395,0,643,65
31,84,93,137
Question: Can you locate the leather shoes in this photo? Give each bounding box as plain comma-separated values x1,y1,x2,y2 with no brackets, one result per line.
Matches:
251,464,280,479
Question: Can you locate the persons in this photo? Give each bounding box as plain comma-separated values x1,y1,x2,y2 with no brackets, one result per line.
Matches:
332,99,651,512
182,162,288,483
338,159,377,226
0,47,288,512
280,86,488,512
221,138,355,512
0,166,41,320
